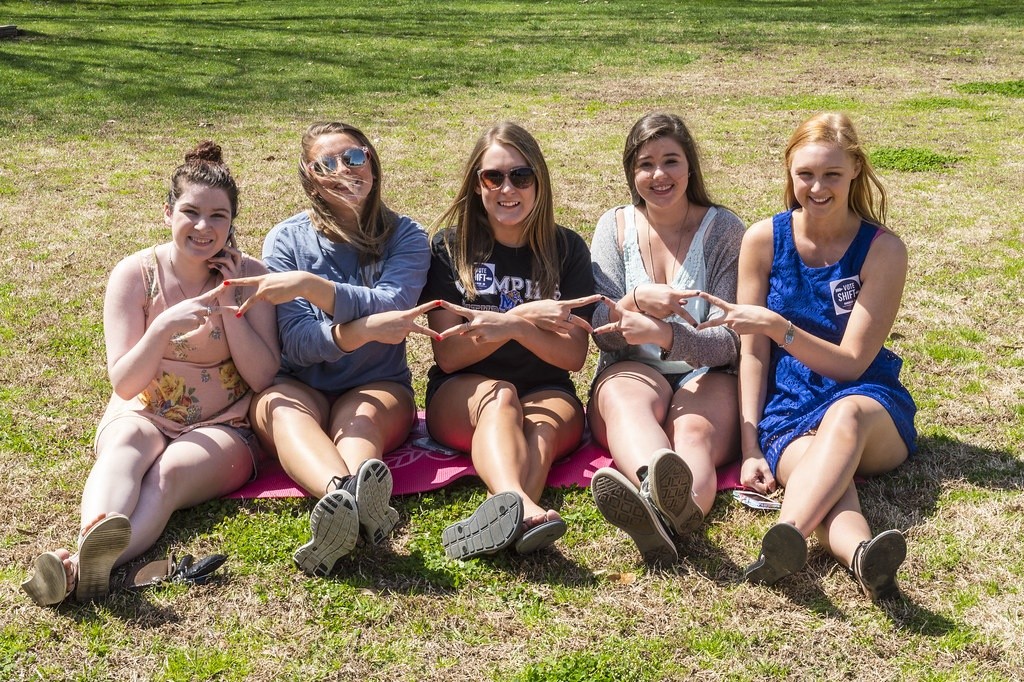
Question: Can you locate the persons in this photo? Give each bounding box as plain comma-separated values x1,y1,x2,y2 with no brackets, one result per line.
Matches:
695,112,919,599
223,121,442,577
416,121,601,561
586,110,748,571
20,139,282,607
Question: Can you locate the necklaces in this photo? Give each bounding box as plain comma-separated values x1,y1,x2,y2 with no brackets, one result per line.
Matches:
169,244,211,299
647,202,689,283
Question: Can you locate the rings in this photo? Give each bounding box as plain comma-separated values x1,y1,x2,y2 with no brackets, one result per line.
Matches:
567,314,572,322
466,322,470,331
207,307,211,315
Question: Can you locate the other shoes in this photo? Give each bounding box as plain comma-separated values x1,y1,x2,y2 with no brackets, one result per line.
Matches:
591,467,680,566
293,490,361,576
745,524,809,588
635,448,707,537
852,528,907,603
331,458,401,548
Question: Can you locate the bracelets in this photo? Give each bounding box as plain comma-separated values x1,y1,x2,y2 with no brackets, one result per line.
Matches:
778,320,795,348
634,286,640,310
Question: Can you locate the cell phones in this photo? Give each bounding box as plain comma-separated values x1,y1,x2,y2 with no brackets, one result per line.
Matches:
733,490,780,510
413,437,457,455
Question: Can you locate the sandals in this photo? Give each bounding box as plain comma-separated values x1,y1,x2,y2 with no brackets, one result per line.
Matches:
21,550,77,608
440,488,523,561
71,511,133,603
519,511,569,557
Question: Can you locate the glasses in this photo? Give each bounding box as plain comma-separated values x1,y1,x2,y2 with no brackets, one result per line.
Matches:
474,167,539,193
308,145,370,178
